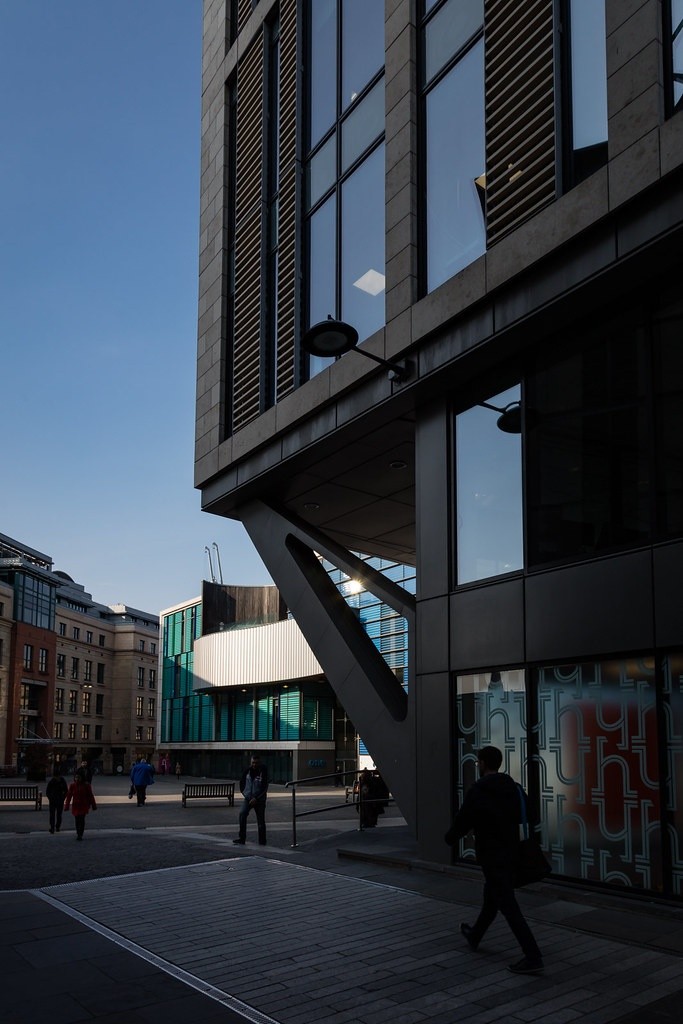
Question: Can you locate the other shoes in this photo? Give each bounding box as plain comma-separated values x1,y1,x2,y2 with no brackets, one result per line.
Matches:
258,840,267,846
56,825,60,832
77,835,83,841
232,839,245,845
49,828,54,834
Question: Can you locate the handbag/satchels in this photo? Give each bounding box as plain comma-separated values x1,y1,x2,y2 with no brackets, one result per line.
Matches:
510,837,553,890
128,784,137,799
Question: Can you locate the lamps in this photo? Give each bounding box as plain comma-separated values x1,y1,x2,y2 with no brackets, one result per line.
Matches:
302,315,414,384
481,401,523,434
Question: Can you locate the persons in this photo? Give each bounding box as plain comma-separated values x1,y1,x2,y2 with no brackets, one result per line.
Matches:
233,754,269,846
130,758,155,807
46,768,69,834
73,759,97,784
459,746,551,975
63,771,98,842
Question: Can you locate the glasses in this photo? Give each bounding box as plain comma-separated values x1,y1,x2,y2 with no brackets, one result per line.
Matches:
474,761,479,767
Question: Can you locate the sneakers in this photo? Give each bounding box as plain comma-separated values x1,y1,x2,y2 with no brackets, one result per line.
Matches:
459,922,480,951
506,955,545,973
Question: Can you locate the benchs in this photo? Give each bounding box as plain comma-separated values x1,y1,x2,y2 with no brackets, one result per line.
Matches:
345,780,359,803
181,783,236,808
0,784,42,811
